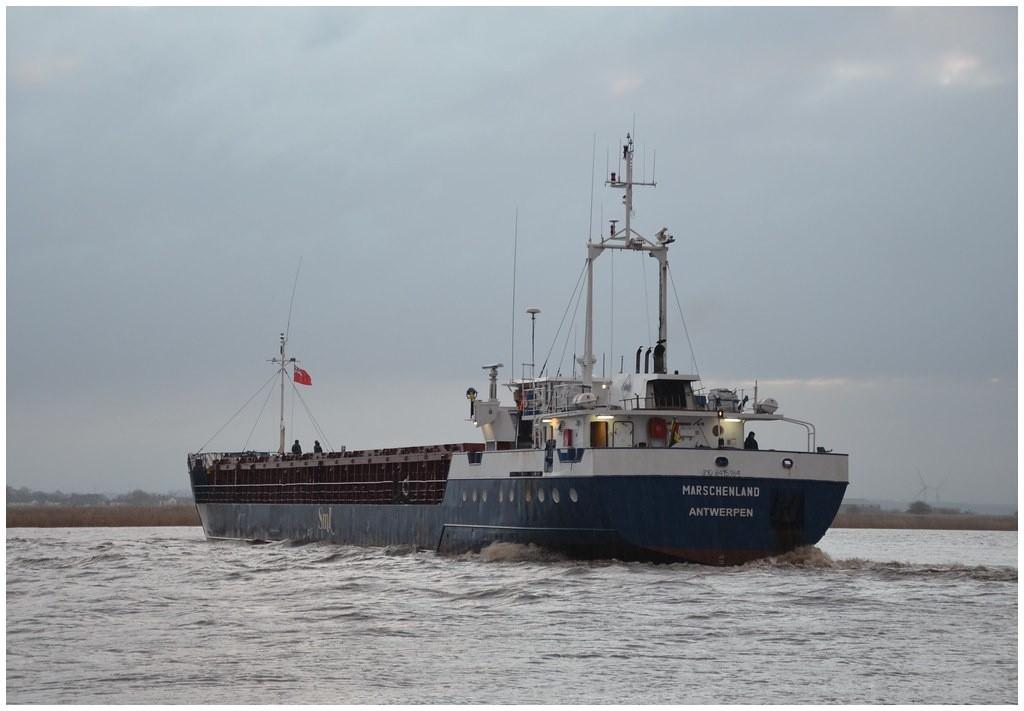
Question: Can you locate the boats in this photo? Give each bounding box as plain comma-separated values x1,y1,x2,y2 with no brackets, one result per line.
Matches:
187,112,849,568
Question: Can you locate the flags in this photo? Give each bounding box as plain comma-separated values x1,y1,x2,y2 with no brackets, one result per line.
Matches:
294,365,312,385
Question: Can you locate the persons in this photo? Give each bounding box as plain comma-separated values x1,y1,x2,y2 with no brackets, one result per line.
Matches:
292,440,302,454
744,431,758,450
313,440,322,452
